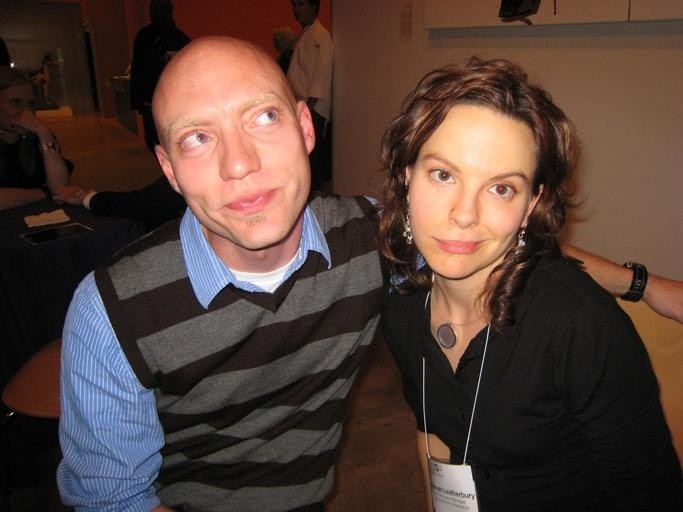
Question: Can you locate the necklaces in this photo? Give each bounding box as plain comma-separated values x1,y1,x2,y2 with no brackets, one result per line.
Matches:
429,285,490,350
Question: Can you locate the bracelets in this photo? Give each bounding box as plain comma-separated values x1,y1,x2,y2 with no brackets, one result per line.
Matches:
620,259,648,304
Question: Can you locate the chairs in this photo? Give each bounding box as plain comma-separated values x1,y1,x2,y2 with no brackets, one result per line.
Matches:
3,295,683,512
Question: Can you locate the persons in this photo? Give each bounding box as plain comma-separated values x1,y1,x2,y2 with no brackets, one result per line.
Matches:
129,1,193,156
29,54,56,107
377,51,680,509
23,165,194,350
267,26,296,76
55,31,682,509
0,66,77,215
283,2,333,177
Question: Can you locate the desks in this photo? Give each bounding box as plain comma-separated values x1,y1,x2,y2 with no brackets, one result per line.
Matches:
1,198,142,385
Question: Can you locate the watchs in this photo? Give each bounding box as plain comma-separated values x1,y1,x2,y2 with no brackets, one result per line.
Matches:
37,141,61,153
